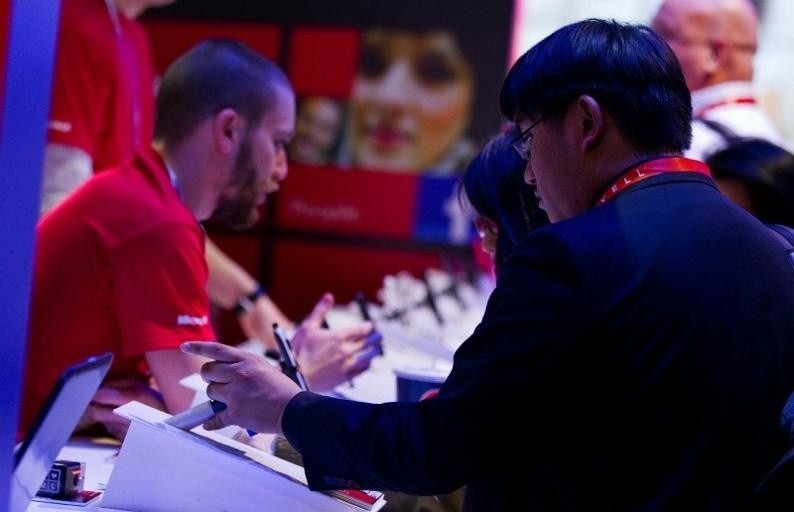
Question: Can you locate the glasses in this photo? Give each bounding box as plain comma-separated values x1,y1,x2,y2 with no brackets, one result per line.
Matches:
508,115,548,161
470,214,503,238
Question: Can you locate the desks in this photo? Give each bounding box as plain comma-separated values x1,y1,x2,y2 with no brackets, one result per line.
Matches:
13,300,488,512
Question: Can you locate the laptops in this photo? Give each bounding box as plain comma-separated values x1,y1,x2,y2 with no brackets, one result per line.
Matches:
8,352,114,512
